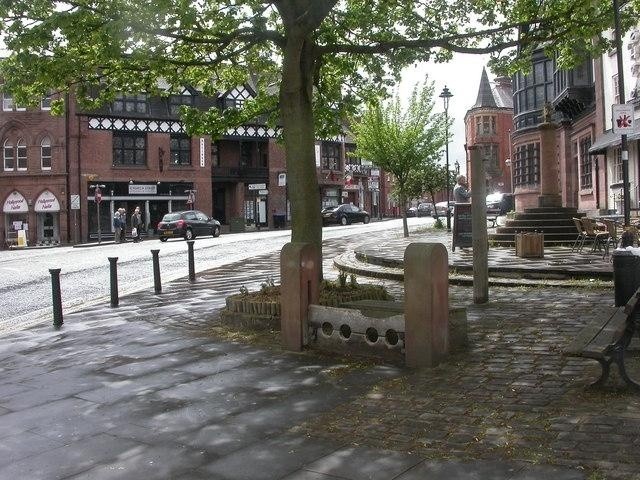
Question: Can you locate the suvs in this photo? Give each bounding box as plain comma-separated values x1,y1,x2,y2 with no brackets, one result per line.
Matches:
157,210,222,242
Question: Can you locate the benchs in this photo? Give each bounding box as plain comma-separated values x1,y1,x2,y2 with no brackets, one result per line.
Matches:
339,298,468,346
561,287,640,390
483,201,505,227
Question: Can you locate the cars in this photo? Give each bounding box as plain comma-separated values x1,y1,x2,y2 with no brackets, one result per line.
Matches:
407,191,513,218
321,204,372,227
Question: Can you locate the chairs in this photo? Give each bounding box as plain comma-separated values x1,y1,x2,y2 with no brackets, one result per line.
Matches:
571,216,640,259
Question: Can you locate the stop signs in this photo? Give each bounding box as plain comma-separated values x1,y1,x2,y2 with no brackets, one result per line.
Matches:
94,188,102,204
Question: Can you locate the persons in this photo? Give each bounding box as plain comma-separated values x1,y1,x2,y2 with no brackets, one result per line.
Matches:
136,206,145,241
453,174,472,249
122,208,128,241
130,209,140,243
118,208,126,242
113,211,122,244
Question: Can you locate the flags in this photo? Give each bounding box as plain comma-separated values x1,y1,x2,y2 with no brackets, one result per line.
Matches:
330,167,336,182
346,170,353,187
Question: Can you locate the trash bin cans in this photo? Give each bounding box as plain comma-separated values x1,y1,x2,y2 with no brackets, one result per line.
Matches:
612,249,640,307
273,214,285,228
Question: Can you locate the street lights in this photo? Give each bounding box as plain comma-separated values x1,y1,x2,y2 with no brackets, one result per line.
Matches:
439,85,454,232
89,182,106,243
183,189,197,210
